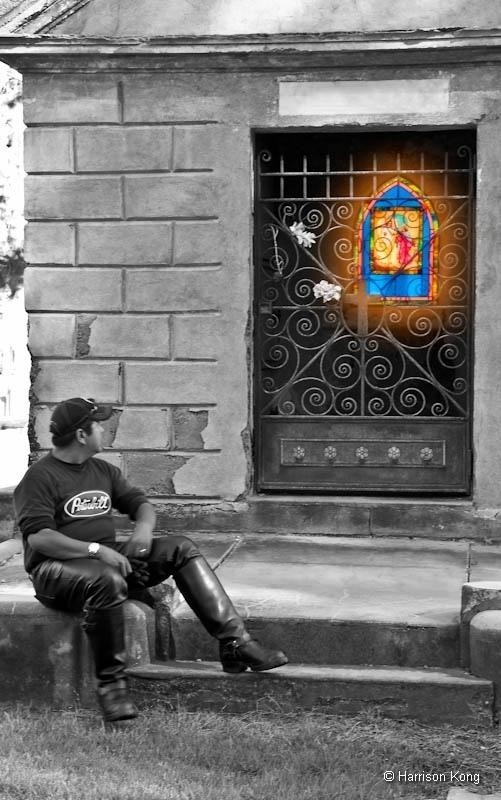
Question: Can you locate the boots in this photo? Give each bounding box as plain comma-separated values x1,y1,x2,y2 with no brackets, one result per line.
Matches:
172,553,289,674
81,604,142,720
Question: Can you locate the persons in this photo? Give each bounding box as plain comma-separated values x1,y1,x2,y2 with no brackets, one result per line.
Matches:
13,398,290,722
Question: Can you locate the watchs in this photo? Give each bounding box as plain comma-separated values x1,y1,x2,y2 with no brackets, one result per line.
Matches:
87,542,99,558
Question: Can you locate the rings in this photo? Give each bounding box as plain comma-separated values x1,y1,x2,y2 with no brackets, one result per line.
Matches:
139,549,148,553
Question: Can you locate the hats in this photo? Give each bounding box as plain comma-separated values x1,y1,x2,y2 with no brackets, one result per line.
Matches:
50,396,113,435
